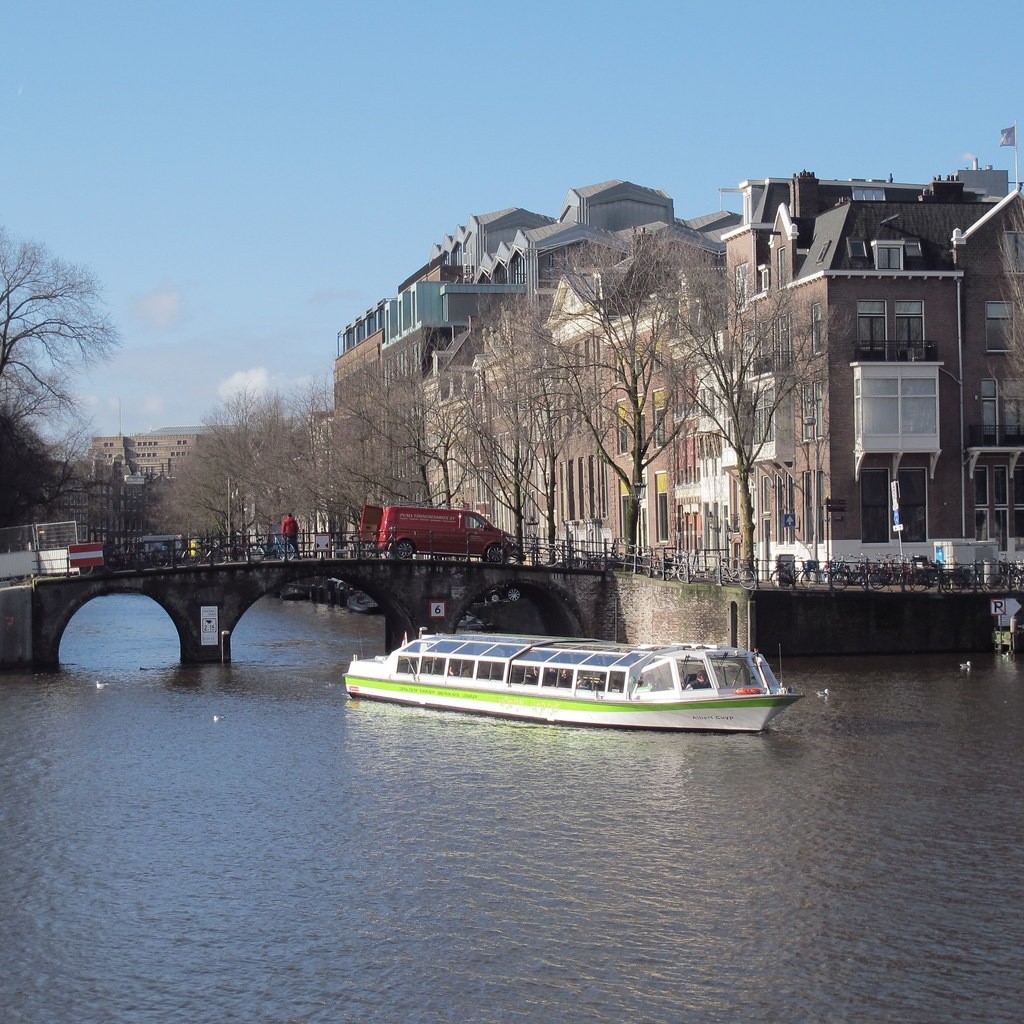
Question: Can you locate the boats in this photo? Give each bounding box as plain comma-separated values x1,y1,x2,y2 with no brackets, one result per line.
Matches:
341,626,806,732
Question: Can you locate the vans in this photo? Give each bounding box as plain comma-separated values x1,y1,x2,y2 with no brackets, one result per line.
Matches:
359,501,518,563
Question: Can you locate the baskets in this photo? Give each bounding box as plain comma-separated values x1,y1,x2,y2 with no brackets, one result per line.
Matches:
740,563,754,571
803,560,817,571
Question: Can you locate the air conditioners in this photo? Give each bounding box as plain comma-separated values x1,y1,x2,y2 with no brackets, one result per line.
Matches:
898,348,914,362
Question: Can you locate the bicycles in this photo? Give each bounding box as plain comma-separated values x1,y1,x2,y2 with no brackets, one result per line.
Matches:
74,522,409,577
496,530,1024,600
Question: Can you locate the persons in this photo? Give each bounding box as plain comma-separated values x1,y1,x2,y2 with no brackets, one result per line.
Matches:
545,668,590,689
282,513,302,560
684,669,711,690
